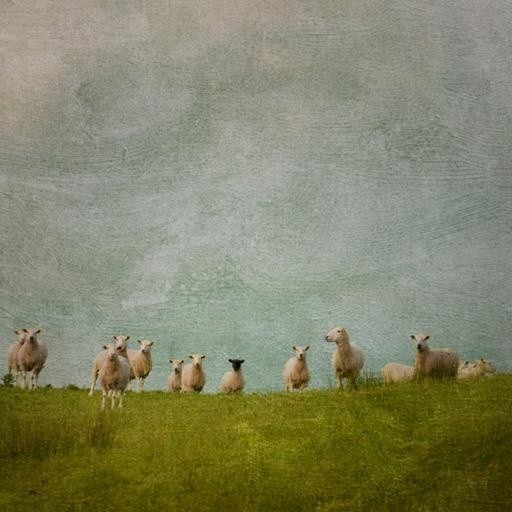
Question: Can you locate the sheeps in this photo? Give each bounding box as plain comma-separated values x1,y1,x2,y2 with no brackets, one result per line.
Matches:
219,358,245,395
87,334,154,411
325,325,365,392
410,333,460,382
7,327,49,393
457,356,497,380
182,353,205,394
380,361,415,385
167,358,184,394
282,345,311,395
